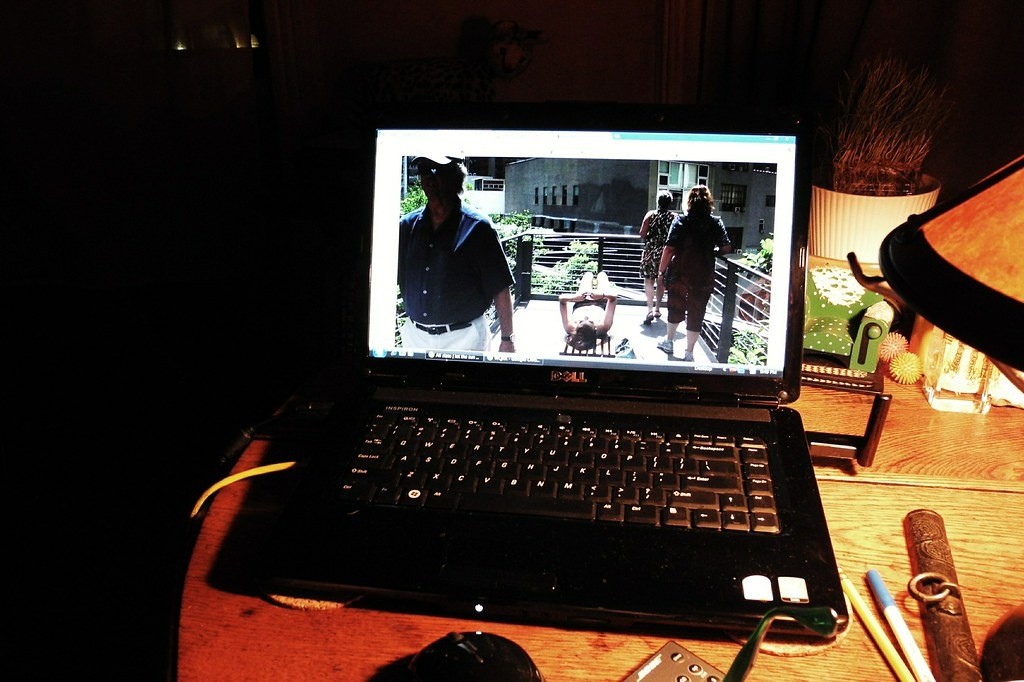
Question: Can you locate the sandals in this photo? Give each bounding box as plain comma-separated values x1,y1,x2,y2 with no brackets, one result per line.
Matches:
654,310,662,318
644,311,654,324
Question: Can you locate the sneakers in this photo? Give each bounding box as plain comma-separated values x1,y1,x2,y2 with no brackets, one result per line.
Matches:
685,349,694,362
657,337,673,353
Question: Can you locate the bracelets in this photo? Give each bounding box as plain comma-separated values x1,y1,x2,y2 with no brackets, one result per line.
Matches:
603,292,605,299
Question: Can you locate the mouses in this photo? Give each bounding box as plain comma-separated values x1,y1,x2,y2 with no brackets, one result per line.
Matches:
407,631,543,682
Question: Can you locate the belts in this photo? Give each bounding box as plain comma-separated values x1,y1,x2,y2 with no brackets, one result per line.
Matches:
409,315,483,335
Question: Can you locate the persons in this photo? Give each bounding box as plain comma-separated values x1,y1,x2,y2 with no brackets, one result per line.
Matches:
561,272,618,351
397,146,517,352
638,186,733,362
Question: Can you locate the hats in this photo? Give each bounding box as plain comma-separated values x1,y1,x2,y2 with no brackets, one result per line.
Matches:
410,155,465,168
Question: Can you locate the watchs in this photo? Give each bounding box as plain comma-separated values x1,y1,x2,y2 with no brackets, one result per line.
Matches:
501,333,515,343
659,271,665,276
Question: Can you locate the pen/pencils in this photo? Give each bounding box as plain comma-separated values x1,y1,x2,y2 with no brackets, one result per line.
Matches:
837,566,938,682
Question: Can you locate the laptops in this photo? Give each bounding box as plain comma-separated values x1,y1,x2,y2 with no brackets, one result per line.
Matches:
266,100,850,631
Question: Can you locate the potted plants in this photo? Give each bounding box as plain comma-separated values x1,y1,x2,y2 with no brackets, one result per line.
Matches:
801,58,940,358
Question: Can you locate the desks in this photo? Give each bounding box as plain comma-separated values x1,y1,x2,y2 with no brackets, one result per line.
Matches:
178,345,1024,682
